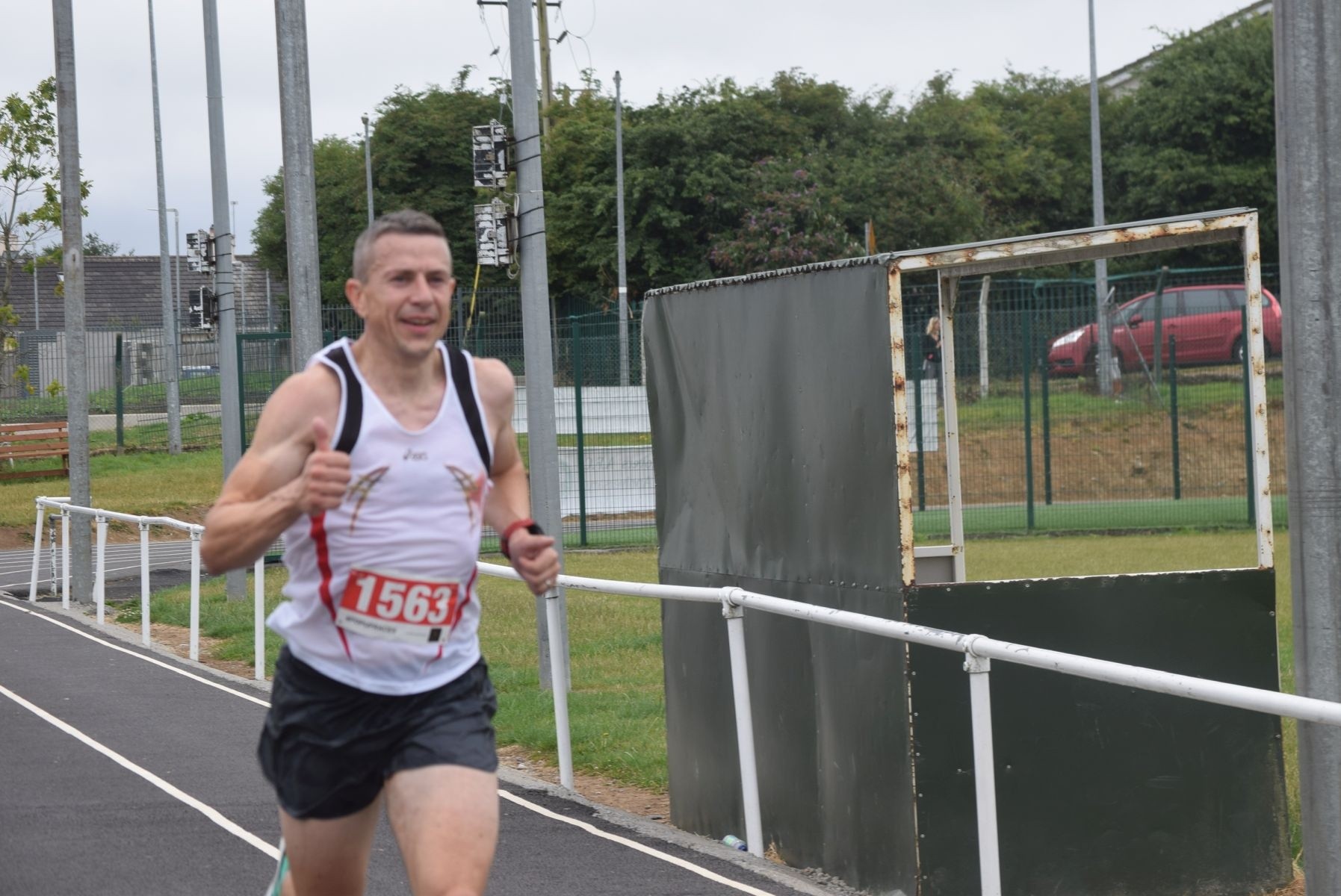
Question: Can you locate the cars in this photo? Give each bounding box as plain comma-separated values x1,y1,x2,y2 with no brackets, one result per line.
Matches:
1042,283,1281,378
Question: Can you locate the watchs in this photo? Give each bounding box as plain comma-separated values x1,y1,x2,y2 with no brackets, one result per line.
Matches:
500,520,544,558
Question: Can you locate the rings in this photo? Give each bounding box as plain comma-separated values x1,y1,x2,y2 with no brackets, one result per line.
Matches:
545,580,552,588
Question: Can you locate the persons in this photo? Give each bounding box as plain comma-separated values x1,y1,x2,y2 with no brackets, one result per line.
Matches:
198,206,561,896
922,316,943,390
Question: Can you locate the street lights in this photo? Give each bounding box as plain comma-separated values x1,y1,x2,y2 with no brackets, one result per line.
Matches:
610,70,631,388
361,116,375,229
147,205,183,381
230,201,237,329
31,240,42,331
